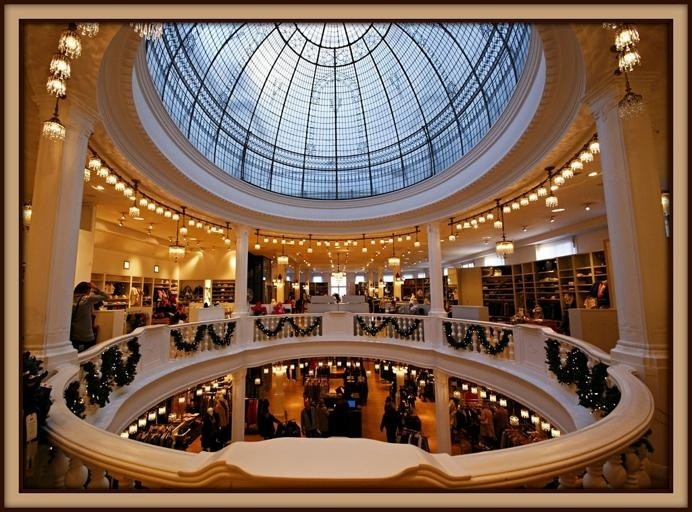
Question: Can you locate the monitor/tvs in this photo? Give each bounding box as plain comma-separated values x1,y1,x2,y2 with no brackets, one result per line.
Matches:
347,400,356,408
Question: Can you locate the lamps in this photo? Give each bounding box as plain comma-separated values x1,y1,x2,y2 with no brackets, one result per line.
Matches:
654,184,670,243
19,198,35,233
83,138,232,265
371,360,561,439
34,20,166,144
448,129,601,259
264,366,269,374
255,378,260,385
252,225,422,268
599,18,646,123
118,371,232,441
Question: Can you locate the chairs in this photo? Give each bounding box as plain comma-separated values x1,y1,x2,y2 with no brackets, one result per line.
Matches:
308,296,339,313
340,294,369,313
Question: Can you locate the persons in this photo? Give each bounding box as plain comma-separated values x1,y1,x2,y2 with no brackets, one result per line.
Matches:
67,281,576,455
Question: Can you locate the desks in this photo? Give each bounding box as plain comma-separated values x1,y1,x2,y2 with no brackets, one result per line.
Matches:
282,357,368,439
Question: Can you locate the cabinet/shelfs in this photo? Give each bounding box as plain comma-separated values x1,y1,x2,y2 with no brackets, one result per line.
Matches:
354,247,611,337
86,268,329,349
